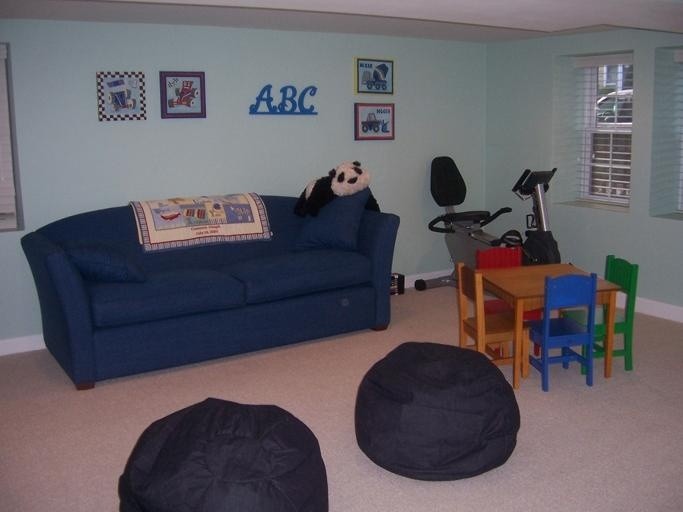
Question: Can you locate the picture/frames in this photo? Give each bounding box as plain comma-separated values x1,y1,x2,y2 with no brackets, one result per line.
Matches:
95,69,147,121
352,54,397,98
159,70,206,120
353,102,395,141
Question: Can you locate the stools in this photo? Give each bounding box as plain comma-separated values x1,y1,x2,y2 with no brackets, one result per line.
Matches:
115,393,330,511
354,342,524,483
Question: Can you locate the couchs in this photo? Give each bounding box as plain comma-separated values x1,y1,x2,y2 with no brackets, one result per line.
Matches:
17,193,400,392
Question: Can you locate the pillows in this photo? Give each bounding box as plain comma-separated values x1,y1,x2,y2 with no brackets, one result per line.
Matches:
299,187,374,254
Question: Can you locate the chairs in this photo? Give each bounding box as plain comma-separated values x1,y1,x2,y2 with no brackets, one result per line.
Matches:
449,246,639,395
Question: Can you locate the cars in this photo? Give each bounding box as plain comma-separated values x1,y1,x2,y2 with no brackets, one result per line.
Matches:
593,88,634,187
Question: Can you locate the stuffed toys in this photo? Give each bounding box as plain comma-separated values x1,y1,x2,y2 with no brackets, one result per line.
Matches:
292,159,381,218
